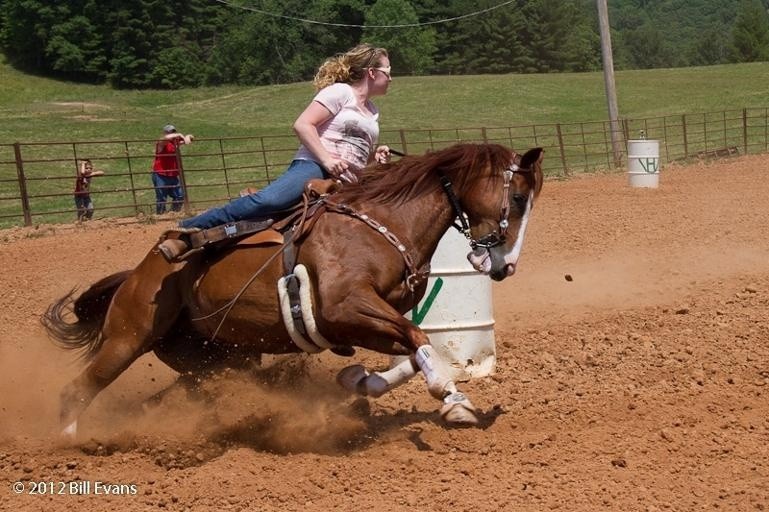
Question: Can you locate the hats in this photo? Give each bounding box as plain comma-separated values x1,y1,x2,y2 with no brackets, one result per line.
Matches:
164,125,176,133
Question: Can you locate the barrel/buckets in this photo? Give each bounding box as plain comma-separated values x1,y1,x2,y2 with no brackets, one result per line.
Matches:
626,139,659,187
404,210,498,379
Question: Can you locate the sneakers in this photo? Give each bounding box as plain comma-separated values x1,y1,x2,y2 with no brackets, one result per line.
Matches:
158,239,190,264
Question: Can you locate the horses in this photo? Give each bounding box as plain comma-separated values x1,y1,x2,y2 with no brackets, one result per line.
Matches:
38,140,545,437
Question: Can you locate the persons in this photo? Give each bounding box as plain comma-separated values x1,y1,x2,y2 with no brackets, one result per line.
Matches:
151,125,194,215
159,44,392,264
73,160,105,221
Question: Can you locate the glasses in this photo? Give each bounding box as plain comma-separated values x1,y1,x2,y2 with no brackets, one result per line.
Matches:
362,65,392,76
